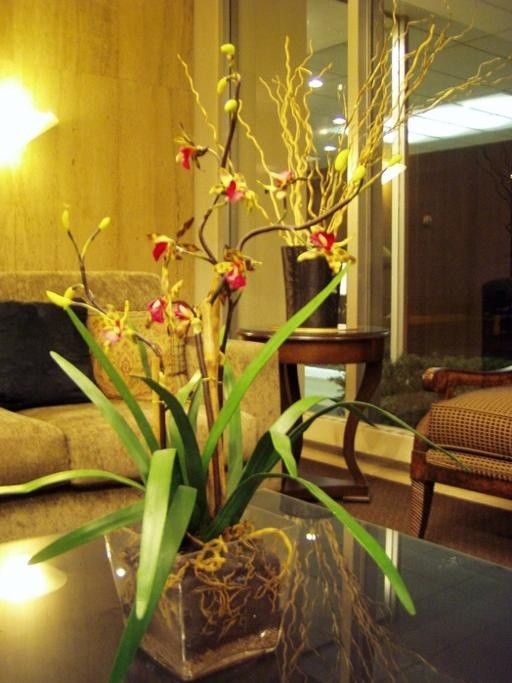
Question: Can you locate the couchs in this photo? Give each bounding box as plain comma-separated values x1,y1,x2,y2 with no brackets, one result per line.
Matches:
0,273,278,500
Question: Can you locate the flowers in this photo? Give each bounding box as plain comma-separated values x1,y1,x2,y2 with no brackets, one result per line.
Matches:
0,42,401,683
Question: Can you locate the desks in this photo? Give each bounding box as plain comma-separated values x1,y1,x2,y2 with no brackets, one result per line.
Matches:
1,482,512,683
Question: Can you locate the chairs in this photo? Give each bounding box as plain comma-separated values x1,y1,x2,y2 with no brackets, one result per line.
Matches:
411,364,511,547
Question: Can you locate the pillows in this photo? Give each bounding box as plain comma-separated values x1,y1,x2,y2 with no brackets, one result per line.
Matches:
0,299,98,409
88,313,190,403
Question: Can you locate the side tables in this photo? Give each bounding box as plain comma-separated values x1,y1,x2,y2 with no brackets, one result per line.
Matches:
238,325,391,504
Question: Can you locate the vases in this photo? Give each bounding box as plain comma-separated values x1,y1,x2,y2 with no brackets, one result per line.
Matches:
104,511,313,683
281,245,340,327
